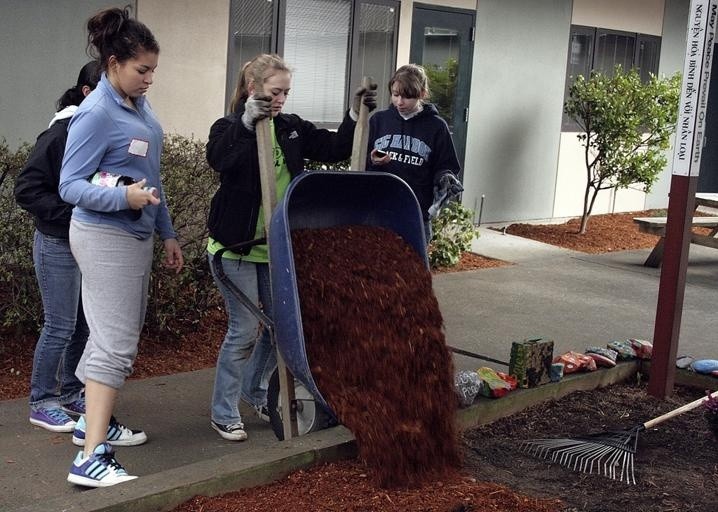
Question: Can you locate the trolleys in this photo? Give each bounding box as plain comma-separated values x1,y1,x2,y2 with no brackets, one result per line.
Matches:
212,76,438,446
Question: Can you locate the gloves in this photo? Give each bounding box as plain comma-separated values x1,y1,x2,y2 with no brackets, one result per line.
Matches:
354,84,377,115
241,93,271,130
428,174,464,216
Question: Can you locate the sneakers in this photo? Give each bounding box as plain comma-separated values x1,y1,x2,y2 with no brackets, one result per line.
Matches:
254,404,270,422
30,408,76,432
62,398,86,415
72,416,147,446
210,420,247,440
67,442,138,487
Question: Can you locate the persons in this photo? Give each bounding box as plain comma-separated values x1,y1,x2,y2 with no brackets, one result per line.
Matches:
206,54,377,443
57,6,184,489
13,59,106,434
367,64,461,246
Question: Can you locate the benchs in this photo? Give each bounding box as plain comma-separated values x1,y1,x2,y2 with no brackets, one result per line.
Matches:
632,214,717,269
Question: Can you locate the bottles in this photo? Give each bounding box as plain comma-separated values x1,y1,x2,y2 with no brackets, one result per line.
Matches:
87,171,158,199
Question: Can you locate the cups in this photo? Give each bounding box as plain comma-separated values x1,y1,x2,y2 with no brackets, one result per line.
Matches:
552,364,563,379
